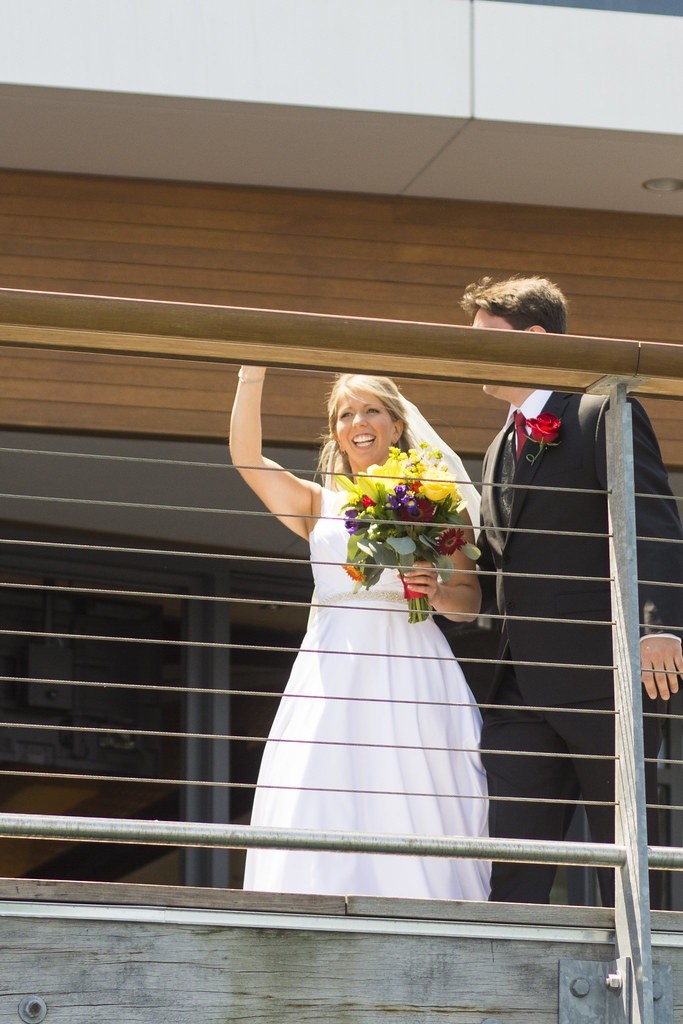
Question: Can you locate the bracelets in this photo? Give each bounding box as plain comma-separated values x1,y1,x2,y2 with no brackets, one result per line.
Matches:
237,370,265,383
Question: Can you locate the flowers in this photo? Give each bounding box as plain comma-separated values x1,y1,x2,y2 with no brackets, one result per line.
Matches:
335,442,481,623
522,412,563,466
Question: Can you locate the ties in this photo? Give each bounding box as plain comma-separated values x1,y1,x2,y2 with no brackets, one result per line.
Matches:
513,411,529,461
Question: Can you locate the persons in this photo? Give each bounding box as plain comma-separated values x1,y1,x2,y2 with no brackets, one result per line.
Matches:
228,364,491,903
459,275,683,911
